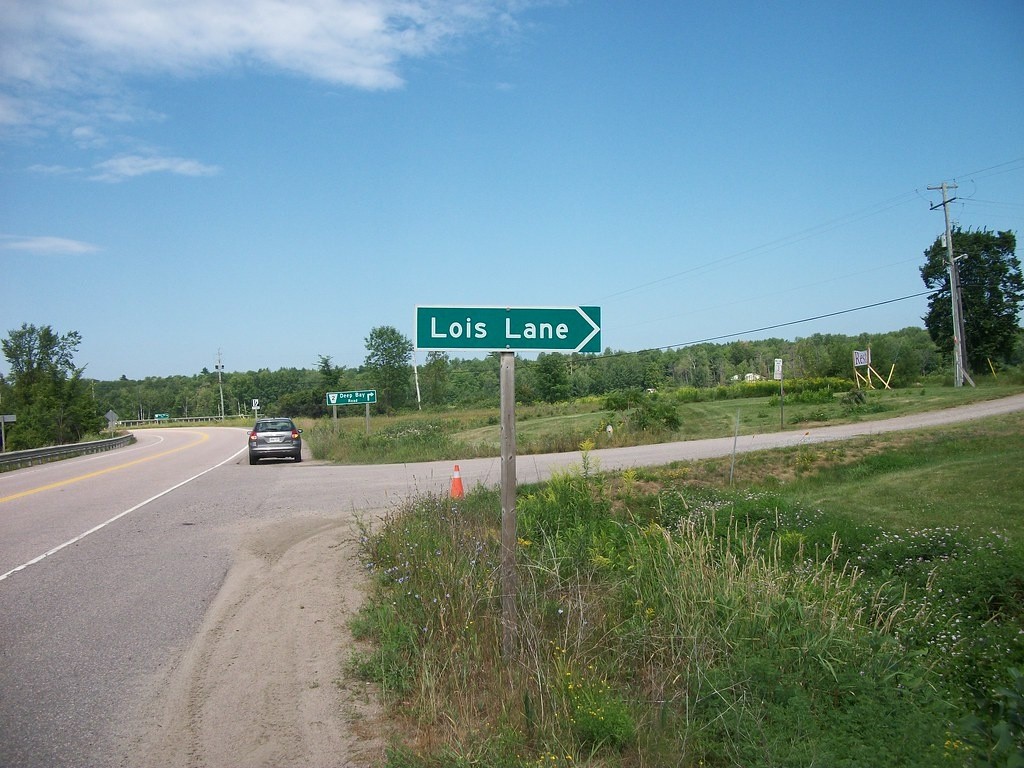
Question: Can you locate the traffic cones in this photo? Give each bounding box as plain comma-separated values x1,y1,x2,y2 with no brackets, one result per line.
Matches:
450,463,465,500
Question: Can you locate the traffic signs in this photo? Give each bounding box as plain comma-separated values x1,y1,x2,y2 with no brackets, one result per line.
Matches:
413,305,604,352
326,390,377,406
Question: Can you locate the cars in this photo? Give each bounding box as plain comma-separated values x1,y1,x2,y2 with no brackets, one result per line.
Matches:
245,419,305,463
